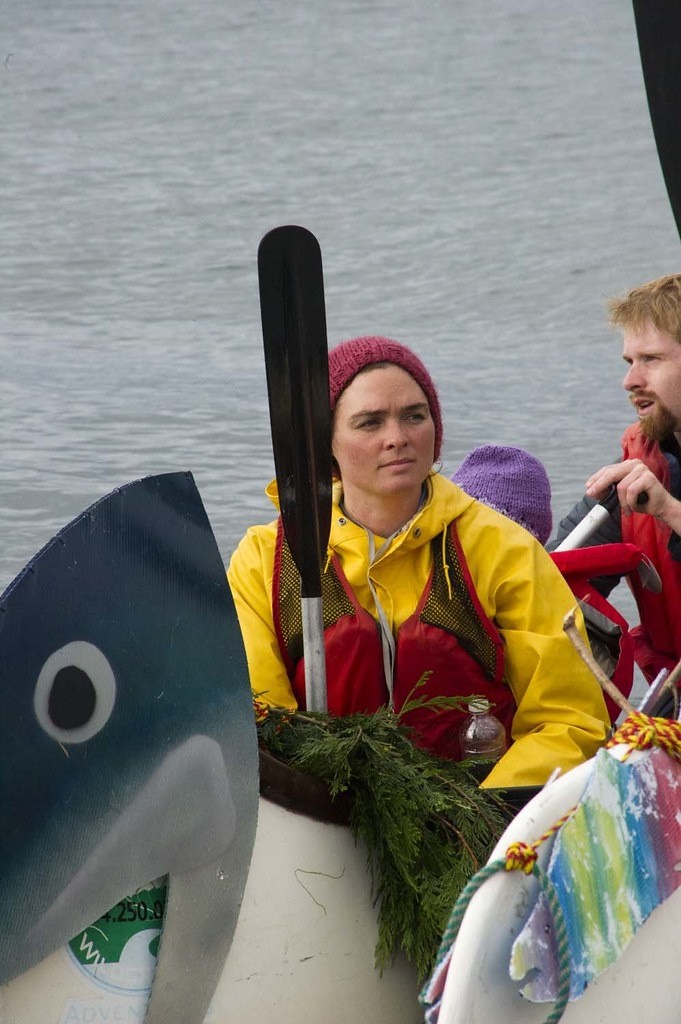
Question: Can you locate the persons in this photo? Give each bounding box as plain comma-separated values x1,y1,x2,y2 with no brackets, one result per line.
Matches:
227,337,612,790
450,444,553,546
545,273,681,685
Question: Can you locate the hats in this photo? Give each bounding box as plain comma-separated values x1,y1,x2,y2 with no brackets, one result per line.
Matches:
448,444,552,546
329,335,443,463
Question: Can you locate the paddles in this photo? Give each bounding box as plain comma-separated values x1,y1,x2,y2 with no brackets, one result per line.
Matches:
255,223,334,713
552,478,644,557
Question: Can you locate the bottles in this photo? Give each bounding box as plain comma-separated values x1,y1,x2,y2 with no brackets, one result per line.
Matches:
459,699,512,794
